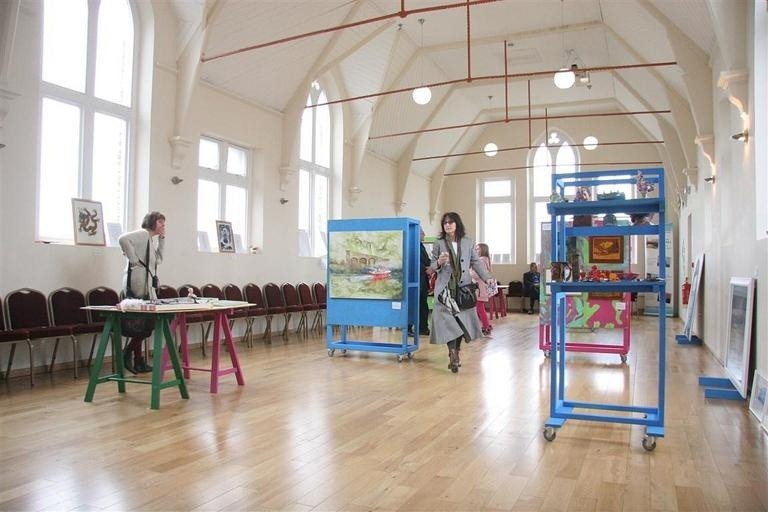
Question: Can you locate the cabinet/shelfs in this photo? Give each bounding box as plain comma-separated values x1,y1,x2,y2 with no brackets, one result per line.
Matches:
542,168,666,451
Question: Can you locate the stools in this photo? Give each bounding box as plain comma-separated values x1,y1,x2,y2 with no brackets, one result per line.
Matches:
490,288,507,319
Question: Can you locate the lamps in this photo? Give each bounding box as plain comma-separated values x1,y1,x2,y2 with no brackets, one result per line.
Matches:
553,0,576,89
582,86,598,150
412,20,432,105
484,95,498,157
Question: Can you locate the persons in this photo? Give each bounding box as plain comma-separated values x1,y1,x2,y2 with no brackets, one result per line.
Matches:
467,243,494,336
428,213,497,371
406,225,432,335
522,262,540,314
118,210,167,376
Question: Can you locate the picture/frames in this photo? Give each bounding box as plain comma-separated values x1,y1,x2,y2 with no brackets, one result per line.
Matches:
588,270,623,300
723,276,755,400
589,236,624,264
72,198,106,246
215,221,235,253
748,369,768,422
758,391,768,433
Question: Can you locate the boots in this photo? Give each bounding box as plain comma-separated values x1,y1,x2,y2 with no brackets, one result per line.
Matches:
125,357,153,374
448,350,460,372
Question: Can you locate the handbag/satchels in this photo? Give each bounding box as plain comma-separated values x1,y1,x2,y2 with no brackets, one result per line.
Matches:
120,290,151,337
457,284,480,309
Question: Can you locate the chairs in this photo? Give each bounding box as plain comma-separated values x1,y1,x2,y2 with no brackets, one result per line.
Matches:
496,280,538,313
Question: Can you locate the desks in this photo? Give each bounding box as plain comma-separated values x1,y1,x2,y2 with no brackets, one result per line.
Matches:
83,296,258,410
